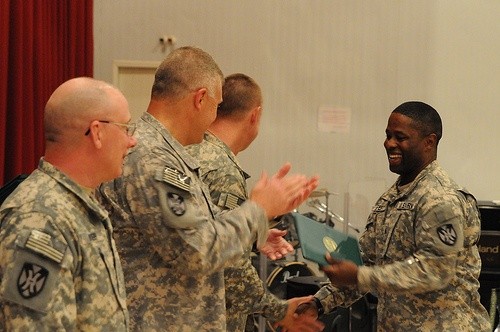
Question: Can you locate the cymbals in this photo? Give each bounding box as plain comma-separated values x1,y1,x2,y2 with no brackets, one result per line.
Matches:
309,189,331,198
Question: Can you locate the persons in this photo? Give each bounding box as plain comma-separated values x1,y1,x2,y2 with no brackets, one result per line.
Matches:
96,47,320,332
0,76,138,332
184,74,325,332
273,102,493,332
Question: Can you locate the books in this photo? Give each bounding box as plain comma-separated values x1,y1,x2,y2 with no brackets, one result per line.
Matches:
293,213,363,268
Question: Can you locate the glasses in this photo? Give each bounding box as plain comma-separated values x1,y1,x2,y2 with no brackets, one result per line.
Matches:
85,120,137,137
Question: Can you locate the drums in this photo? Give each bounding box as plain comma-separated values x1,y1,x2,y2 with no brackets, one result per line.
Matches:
253,262,316,332
